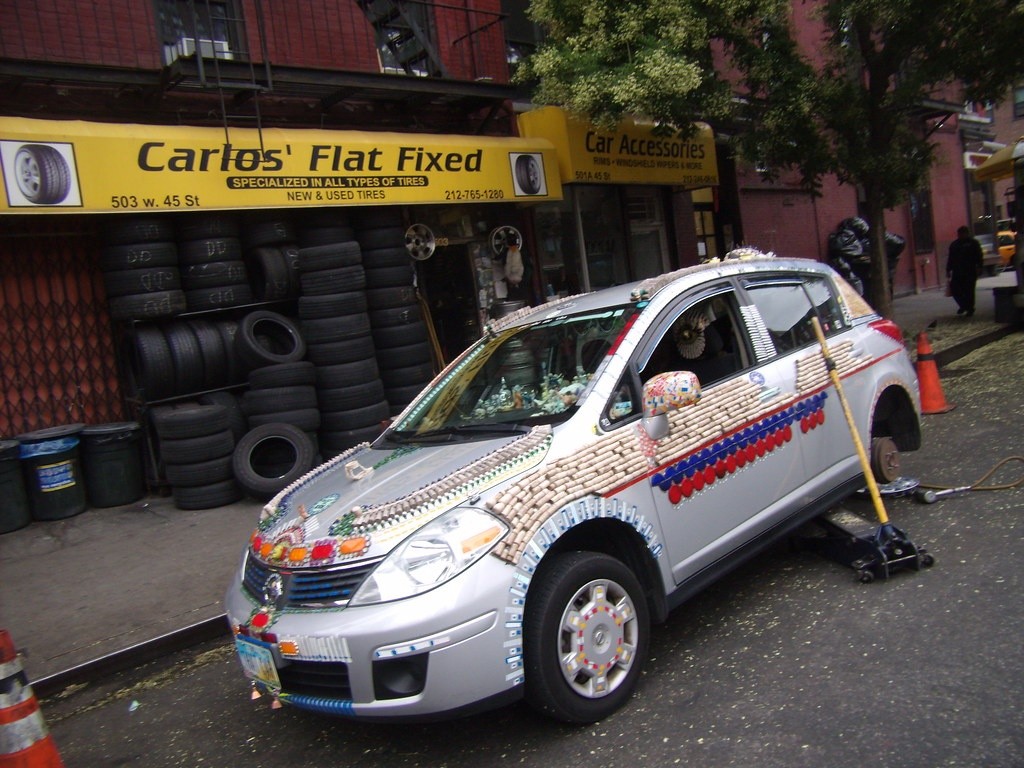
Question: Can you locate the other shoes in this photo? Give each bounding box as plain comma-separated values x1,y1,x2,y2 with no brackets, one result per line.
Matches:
957,308,967,314
966,309,976,316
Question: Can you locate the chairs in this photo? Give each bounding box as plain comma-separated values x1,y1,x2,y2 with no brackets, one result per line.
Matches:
562,338,629,391
654,324,737,387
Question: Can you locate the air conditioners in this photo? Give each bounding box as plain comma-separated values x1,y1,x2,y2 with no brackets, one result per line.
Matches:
177,37,231,60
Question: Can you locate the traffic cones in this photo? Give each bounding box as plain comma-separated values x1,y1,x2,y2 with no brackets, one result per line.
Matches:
917,330,956,416
0,628,62,767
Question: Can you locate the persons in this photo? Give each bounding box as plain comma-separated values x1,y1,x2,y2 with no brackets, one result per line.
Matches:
945,226,986,317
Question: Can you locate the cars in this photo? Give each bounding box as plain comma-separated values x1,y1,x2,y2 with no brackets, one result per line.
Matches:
999,228,1017,272
228,251,924,718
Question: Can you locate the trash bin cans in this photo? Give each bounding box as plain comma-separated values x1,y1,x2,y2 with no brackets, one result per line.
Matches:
83,418,146,511
0,438,31,536
17,423,90,522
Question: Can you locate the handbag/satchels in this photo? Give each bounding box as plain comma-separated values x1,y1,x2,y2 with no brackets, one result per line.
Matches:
944,277,952,297
